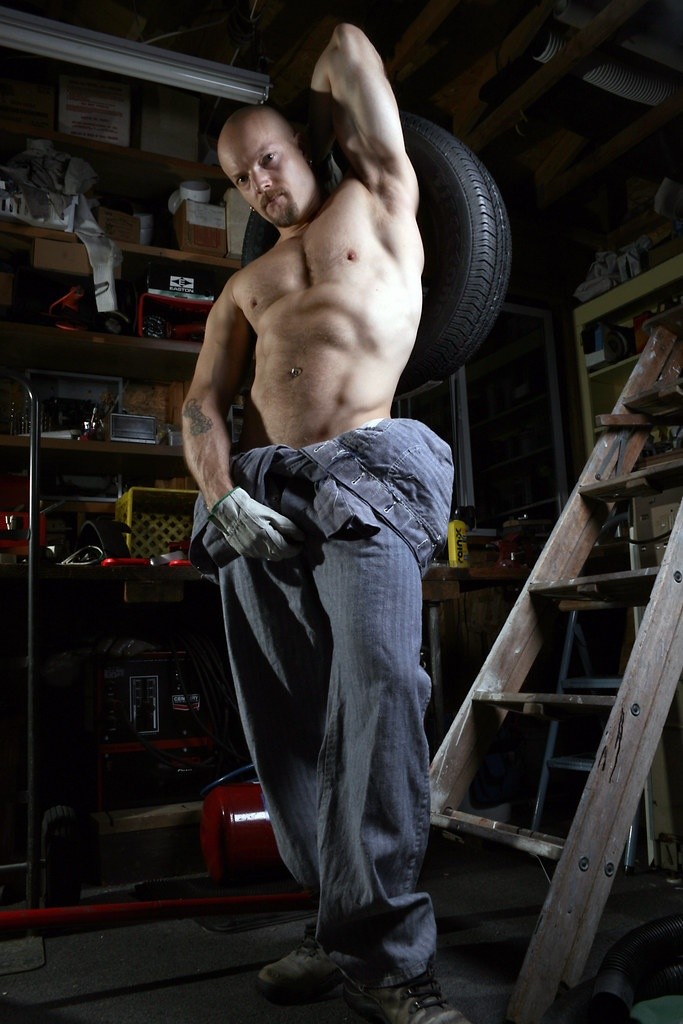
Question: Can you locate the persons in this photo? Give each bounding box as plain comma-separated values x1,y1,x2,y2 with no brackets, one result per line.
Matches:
179,23,473,1024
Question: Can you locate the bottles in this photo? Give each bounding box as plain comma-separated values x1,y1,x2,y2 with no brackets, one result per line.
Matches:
447,508,470,569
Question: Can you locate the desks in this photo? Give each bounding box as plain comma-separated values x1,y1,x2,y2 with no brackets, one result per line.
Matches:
420,567,531,842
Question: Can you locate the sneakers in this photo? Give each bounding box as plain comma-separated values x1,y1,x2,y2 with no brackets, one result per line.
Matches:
346,960,474,1024
256,924,344,1004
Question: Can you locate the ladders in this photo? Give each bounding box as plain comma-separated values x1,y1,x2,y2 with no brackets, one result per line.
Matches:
426,301,683,1024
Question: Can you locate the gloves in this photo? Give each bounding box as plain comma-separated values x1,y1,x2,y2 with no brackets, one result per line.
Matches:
206,486,303,562
300,124,343,203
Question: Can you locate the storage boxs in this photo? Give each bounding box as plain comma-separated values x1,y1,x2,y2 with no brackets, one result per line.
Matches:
55,62,129,147
127,293,213,345
129,80,200,162
168,198,228,258
90,206,141,244
0,79,54,131
116,486,200,558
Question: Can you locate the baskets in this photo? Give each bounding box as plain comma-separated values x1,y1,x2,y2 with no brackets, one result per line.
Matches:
115,486,199,563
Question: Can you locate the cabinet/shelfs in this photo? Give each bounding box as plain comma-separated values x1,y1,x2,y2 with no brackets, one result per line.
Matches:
573,253,683,880
0,67,281,937
